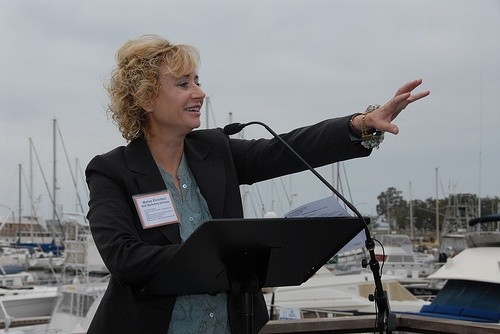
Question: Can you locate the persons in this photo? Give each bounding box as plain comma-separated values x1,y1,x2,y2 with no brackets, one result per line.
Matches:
82,30,432,334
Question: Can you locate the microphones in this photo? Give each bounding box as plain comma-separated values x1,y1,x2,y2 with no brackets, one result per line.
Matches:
223,121,371,242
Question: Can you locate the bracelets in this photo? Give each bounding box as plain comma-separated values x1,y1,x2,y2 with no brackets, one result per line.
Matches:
360,103,385,149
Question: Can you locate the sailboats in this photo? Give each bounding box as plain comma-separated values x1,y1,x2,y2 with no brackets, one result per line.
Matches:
0,96,500,333
